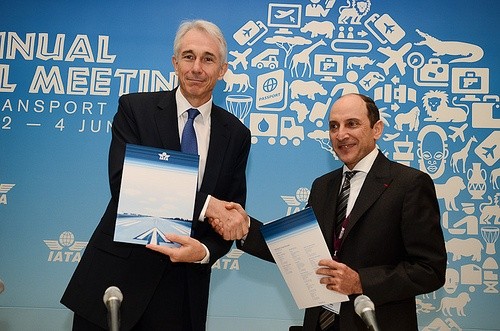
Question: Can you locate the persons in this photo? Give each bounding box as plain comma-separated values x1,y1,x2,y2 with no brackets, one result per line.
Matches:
207,93,450,331
58,17,252,331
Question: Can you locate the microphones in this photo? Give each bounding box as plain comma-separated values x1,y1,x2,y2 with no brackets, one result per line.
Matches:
103,286,124,331
354,295,381,331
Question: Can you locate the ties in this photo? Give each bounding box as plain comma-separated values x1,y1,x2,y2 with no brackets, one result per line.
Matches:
319,172,349,331
181,109,201,155
